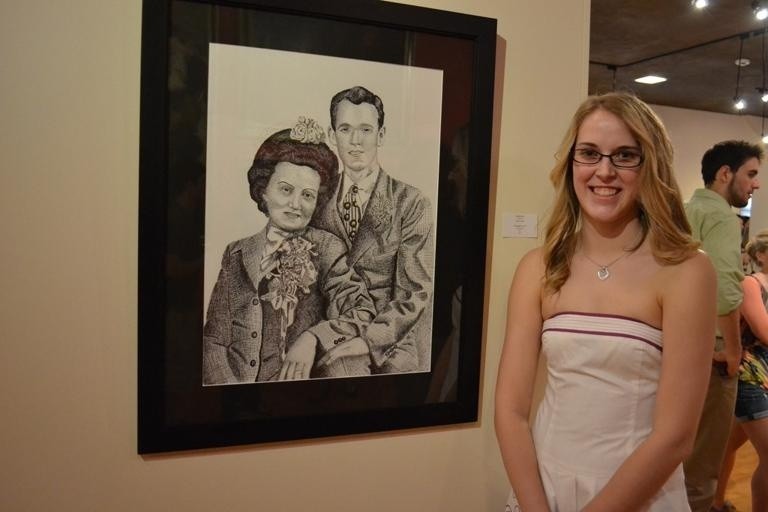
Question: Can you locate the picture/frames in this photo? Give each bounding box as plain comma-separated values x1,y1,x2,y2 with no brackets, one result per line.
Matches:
135,0,498,458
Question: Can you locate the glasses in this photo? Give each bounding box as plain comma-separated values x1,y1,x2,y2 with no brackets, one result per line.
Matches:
569,146,644,169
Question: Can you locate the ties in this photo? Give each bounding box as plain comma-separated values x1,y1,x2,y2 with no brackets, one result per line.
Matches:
341,184,363,244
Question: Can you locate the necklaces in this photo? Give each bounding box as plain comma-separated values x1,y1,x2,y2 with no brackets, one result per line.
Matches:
582,225,644,282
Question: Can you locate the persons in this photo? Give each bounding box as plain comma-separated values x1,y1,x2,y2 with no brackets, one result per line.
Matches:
201,123,380,383
680,137,762,512
710,231,768,512
495,90,719,512
314,85,436,379
